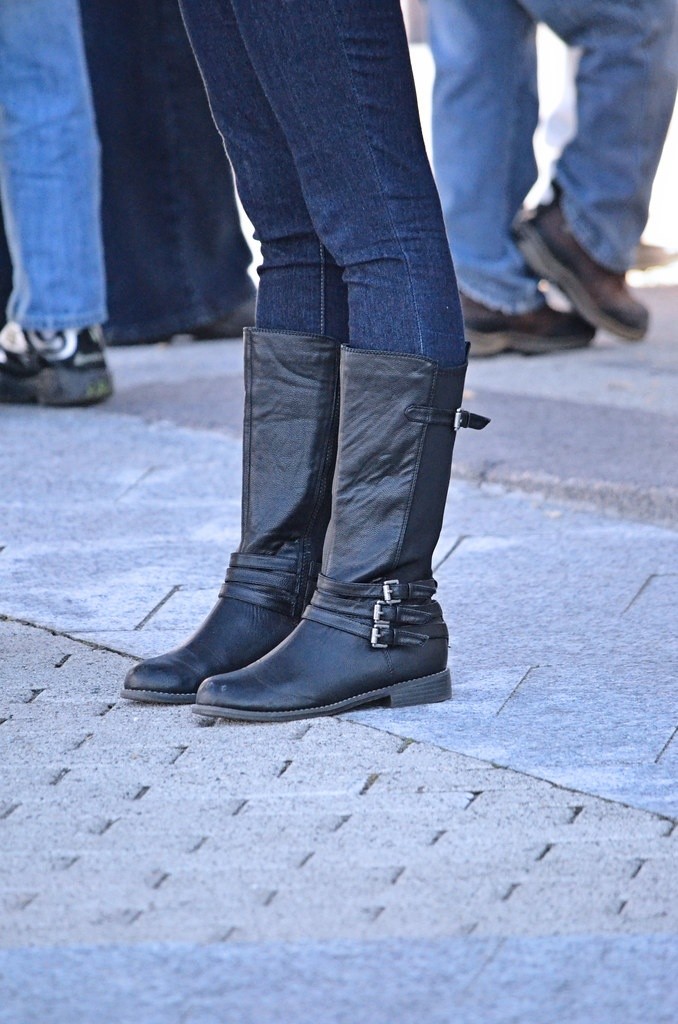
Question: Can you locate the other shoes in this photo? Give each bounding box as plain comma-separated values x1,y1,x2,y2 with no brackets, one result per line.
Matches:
457,290,596,357
159,291,257,343
510,195,650,341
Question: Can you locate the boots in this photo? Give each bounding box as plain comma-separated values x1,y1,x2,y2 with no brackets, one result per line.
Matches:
121,327,471,722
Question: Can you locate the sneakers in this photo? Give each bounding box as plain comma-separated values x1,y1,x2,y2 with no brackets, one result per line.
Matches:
0,321,113,408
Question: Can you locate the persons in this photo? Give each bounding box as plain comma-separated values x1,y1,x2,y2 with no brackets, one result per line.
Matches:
0,0,257,405
121,0,490,722
407,0,678,356
